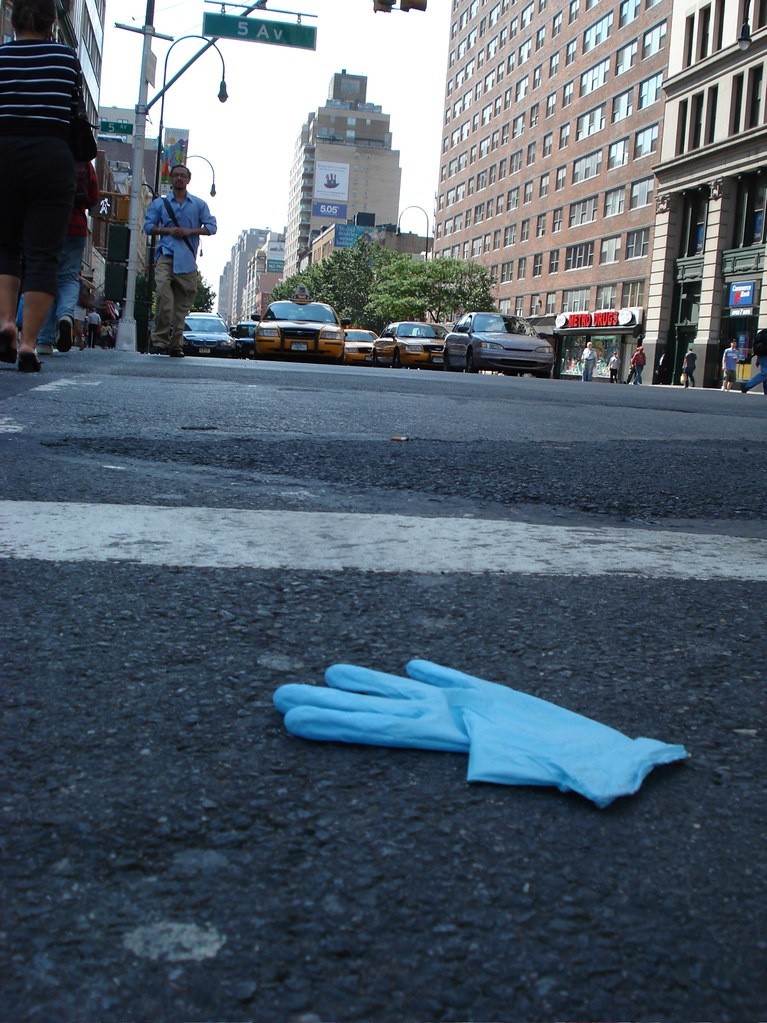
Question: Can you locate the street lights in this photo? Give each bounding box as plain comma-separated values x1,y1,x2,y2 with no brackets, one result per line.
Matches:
140,34,229,355
394,205,430,263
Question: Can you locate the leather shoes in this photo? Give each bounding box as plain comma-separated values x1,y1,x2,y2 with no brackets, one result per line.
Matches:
170,351,185,357
150,347,163,354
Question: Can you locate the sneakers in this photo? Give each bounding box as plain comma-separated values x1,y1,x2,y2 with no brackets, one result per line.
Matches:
37,344,53,354
721,389,725,392
741,383,749,393
56,316,74,353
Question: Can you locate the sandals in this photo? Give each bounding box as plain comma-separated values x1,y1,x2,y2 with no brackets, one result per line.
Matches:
0,330,17,364
19,351,45,372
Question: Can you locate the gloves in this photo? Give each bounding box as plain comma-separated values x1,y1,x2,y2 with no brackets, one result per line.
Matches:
274,660,693,813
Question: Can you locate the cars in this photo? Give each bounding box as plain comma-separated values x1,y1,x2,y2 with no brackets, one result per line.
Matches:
442,312,554,379
187,309,237,338
343,329,379,367
183,316,237,358
234,321,261,359
427,323,455,339
251,281,352,365
370,321,449,369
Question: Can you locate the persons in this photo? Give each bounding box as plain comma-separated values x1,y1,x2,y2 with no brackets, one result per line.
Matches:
659,350,667,384
608,352,619,383
740,328,767,395
87,307,101,350
100,322,117,349
581,342,597,382
0,0,83,372
143,165,217,357
721,339,739,392
36,162,100,354
627,346,646,384
682,348,697,388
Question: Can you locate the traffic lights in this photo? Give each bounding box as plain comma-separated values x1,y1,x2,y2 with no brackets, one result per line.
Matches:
373,0,396,12
400,0,427,12
87,193,113,220
